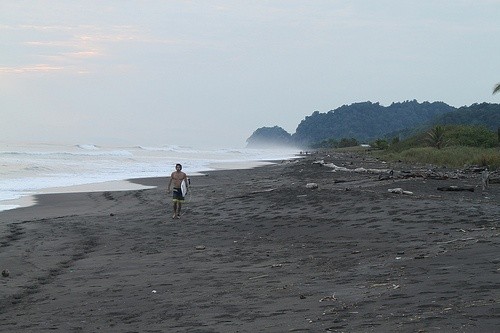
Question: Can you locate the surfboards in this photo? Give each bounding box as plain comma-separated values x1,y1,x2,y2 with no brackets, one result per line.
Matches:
181,177,191,197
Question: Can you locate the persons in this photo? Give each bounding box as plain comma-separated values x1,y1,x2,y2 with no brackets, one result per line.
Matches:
167,163,189,220
